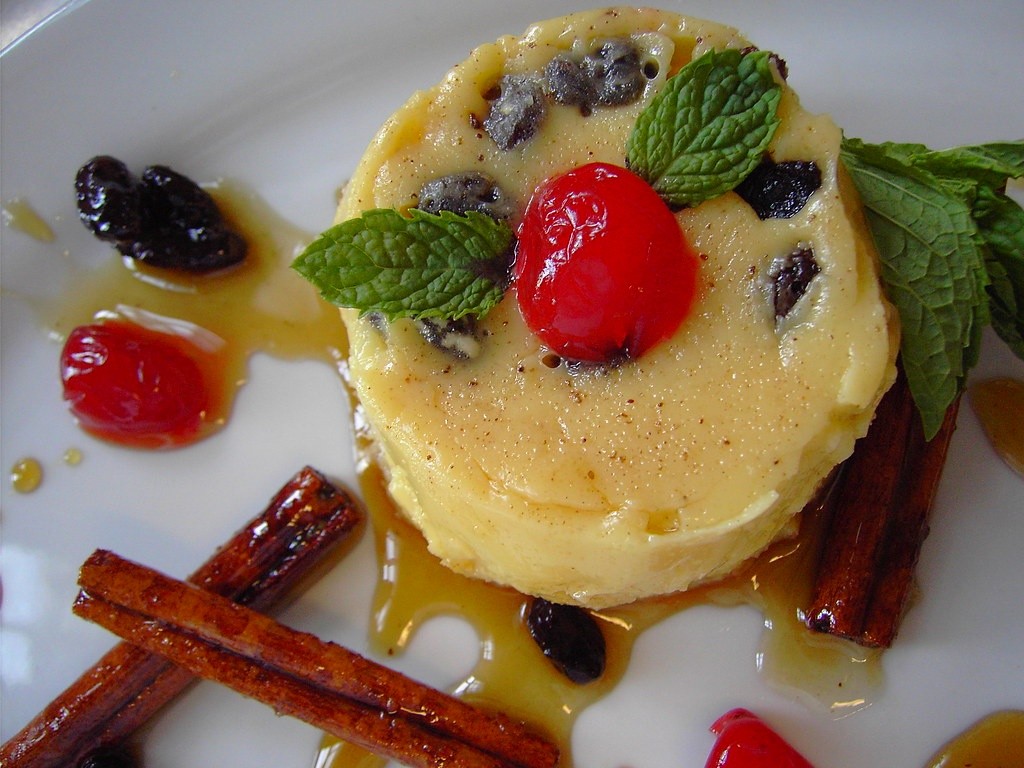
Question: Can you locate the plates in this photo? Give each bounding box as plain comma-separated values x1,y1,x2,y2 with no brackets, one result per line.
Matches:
0,0,1023,768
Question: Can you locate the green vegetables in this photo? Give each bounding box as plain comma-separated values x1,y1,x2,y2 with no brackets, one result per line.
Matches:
833,120,1024,443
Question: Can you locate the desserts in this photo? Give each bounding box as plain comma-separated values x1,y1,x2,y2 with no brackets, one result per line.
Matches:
289,0,903,618
